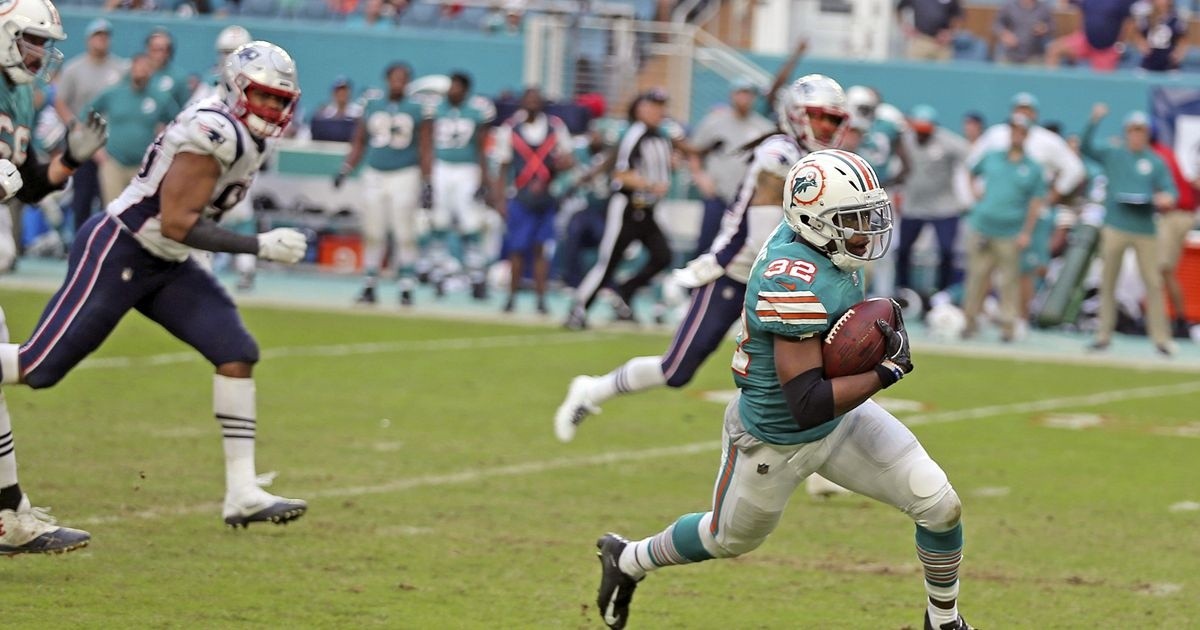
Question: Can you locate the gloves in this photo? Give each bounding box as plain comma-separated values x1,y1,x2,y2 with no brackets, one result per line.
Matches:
256,227,305,265
60,110,113,170
657,253,723,307
0,158,23,203
876,295,915,388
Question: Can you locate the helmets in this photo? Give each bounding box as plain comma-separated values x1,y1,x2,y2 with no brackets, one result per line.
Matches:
212,21,248,54
221,40,299,137
847,86,880,127
780,150,888,272
0,0,63,84
777,72,847,150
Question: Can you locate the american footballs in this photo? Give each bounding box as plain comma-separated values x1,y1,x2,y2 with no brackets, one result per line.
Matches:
823,295,895,384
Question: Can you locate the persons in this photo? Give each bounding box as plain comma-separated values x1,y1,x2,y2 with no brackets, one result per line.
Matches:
1,1,1200,357
594,147,976,630
0,1,112,554
0,39,310,524
554,73,853,492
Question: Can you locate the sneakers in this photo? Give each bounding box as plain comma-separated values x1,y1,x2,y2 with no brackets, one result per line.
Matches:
596,530,645,629
222,485,309,532
0,493,94,555
924,608,978,630
553,375,597,441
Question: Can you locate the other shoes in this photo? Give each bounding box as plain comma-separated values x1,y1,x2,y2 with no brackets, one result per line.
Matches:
357,252,650,334
900,273,1196,355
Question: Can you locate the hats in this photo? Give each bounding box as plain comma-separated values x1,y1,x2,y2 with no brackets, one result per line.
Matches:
1122,110,1149,128
910,101,938,133
1005,113,1031,128
642,87,670,101
84,18,114,39
1013,94,1040,111
